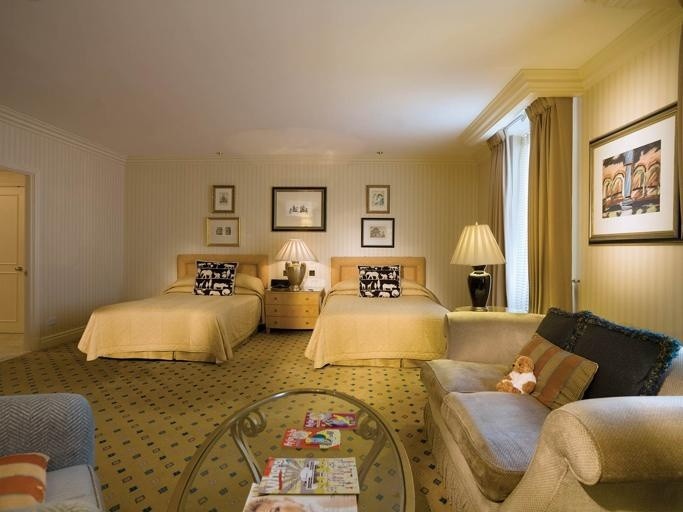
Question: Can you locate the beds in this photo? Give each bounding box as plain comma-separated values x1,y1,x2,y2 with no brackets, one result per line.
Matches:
76,253,269,365
302,255,448,370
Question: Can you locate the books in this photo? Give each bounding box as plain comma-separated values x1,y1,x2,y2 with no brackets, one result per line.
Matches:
282,429,341,449
257,457,361,495
243,483,359,512
303,412,358,430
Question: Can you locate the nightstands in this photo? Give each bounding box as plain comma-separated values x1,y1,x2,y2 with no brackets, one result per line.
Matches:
265,285,325,333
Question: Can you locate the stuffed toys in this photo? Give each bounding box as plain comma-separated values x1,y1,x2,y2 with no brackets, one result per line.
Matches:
496,355,536,394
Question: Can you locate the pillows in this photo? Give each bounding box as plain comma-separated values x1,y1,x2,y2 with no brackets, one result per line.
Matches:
356,264,401,299
563,313,682,403
501,330,599,411
0,450,49,512
191,259,240,298
529,303,592,352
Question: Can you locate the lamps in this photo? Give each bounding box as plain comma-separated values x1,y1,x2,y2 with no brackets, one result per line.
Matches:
278,238,319,292
448,221,506,312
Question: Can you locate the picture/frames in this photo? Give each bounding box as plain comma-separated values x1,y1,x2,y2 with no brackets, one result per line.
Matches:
585,96,682,247
211,184,235,214
360,217,395,248
206,216,240,248
270,186,327,232
364,184,390,214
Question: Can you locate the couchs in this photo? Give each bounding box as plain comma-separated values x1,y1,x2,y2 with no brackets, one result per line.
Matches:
0,393,106,512
421,310,683,512
